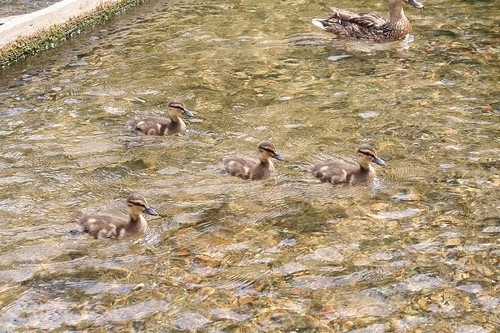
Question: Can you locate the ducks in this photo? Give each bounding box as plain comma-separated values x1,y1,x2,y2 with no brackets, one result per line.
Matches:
69,194,159,242
220,139,284,182
124,99,195,138
311,0,425,44
310,144,388,188
